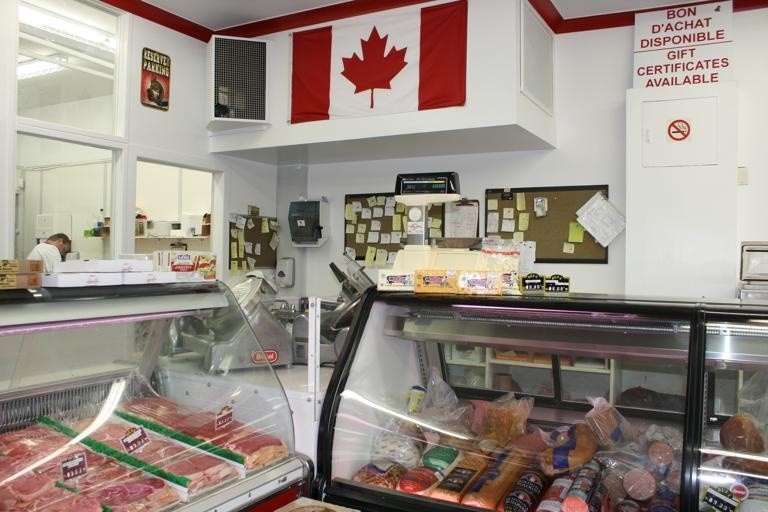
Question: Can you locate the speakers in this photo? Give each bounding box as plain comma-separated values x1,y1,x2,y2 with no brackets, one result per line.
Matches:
205,35,266,130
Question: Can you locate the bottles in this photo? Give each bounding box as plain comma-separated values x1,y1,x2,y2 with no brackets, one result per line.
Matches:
153,251,171,271
96,209,106,227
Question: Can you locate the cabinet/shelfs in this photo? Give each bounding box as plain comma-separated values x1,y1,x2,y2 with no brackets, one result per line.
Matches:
200,1,562,152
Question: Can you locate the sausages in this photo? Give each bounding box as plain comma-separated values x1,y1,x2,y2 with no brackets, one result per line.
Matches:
353,385,680,512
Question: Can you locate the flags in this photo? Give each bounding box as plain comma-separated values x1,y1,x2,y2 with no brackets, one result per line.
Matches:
291,0,468,125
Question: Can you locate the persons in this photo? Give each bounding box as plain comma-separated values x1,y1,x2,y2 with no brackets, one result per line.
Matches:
27,232,71,274
